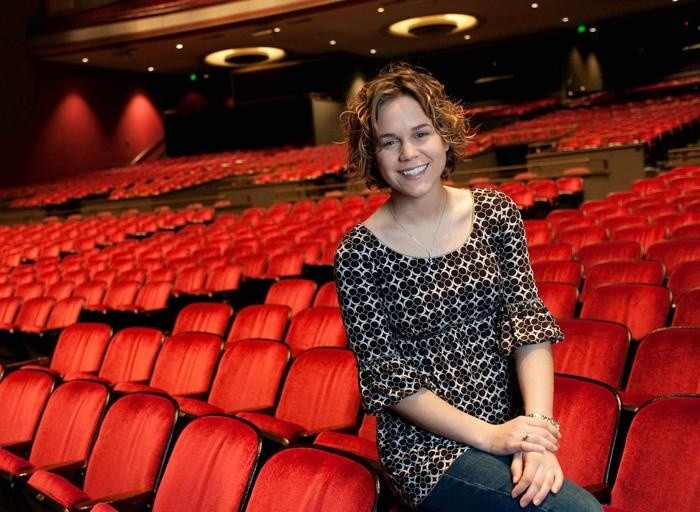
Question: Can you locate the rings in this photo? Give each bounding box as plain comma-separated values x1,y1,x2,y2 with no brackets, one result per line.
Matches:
522,431,532,441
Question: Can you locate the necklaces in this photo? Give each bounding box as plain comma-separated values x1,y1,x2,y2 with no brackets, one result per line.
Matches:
390,187,448,265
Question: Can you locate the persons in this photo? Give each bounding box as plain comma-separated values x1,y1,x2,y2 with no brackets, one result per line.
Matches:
334,62,604,512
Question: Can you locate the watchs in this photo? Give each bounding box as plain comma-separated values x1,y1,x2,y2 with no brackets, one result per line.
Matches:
526,413,559,431
